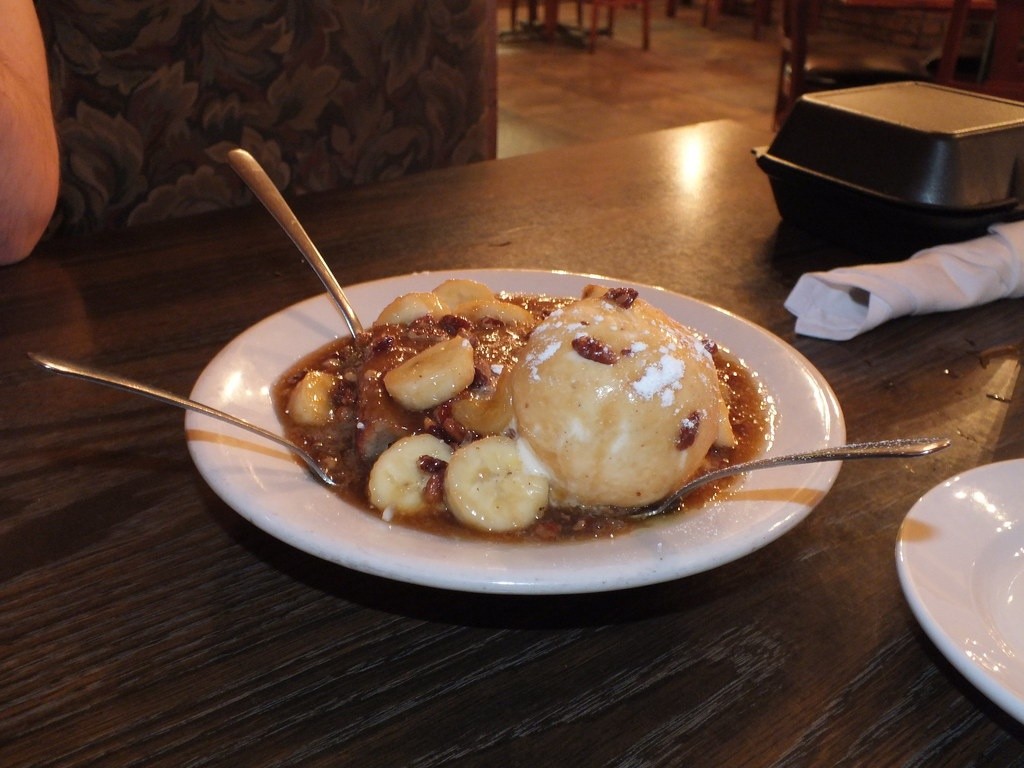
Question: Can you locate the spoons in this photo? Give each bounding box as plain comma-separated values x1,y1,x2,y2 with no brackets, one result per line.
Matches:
24,350,349,492
612,437,951,521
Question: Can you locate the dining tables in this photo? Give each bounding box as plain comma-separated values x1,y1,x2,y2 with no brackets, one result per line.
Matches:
0,117,1024,768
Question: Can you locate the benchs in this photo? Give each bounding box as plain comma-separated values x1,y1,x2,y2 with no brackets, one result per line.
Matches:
35,0,497,246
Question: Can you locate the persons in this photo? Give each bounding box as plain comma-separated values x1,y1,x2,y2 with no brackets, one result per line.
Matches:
0,0,59,264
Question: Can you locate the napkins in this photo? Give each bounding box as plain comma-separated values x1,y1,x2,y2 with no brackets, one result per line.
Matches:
781,222,1024,340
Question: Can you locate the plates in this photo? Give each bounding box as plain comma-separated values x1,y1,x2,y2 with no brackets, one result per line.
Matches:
896,454,1024,730
183,268,848,596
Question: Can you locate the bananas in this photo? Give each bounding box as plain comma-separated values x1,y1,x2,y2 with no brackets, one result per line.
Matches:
294,276,551,529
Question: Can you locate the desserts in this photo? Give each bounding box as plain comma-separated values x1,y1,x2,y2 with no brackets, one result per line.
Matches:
509,283,722,511
